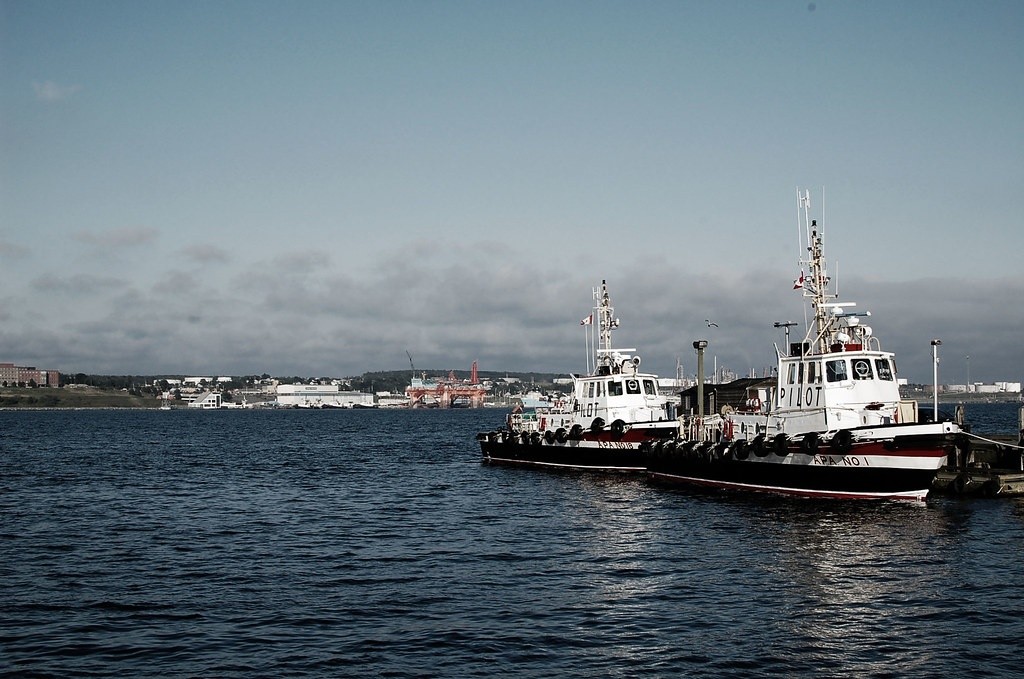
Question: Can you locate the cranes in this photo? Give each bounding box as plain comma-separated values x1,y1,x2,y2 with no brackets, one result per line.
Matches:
405,349,416,380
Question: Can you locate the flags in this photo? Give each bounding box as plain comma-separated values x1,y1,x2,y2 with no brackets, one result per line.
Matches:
793,271,803,289
581,315,592,325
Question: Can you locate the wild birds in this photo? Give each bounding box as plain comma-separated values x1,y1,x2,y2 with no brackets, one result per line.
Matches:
704,318,720,330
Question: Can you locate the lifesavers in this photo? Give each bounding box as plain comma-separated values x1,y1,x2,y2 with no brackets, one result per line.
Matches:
802,432,819,455
611,419,625,438
591,417,605,433
752,436,770,457
571,424,582,440
521,431,530,445
733,439,750,459
773,433,791,456
531,432,542,446
555,428,567,443
830,430,851,453
545,430,554,444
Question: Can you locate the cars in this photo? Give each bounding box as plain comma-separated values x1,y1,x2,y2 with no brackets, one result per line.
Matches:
918,406,971,440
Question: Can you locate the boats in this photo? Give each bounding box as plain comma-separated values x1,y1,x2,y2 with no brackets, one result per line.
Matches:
636,184,964,508
471,279,689,474
